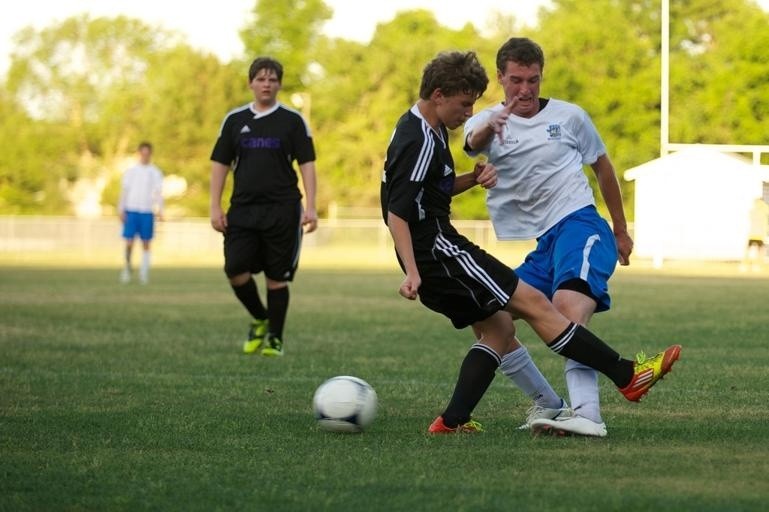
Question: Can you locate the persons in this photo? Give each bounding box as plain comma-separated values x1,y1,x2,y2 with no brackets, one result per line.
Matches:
118,142,164,283
211,57,318,355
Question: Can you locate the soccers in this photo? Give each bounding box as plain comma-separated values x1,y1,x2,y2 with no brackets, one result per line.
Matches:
312,376,377,433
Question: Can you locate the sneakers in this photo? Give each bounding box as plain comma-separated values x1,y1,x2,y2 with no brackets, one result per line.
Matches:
618,343,682,403
427,415,485,435
515,398,608,439
243,318,285,360
120,267,149,283
